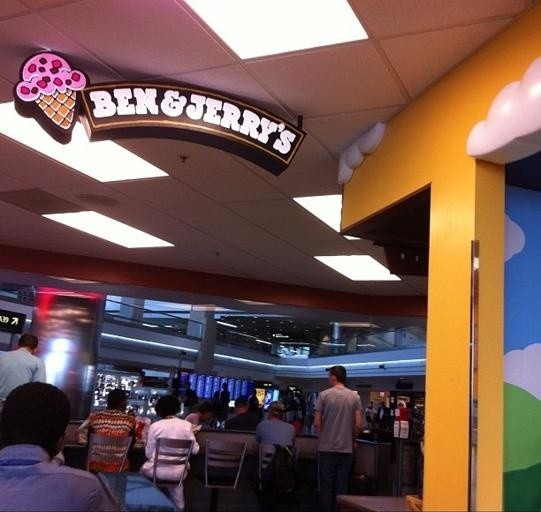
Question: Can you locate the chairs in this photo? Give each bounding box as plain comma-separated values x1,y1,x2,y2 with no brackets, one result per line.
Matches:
83,433,319,512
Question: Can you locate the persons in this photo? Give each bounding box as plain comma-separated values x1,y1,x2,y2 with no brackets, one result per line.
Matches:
78,389,137,474
283,390,389,437
254,401,297,474
0,382,105,512
0,333,44,417
312,365,364,512
179,380,259,431
139,395,200,512
